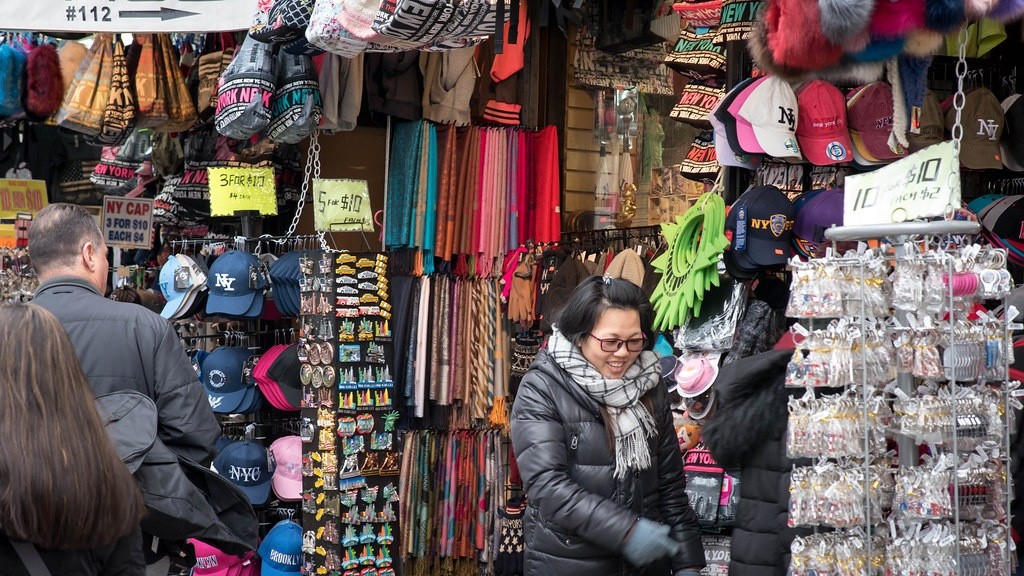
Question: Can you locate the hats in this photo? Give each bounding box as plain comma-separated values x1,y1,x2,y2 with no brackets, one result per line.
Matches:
707,65,1024,280
151,246,305,576
543,248,653,319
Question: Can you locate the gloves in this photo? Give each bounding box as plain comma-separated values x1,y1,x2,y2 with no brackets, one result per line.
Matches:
619,518,677,567
500,244,566,330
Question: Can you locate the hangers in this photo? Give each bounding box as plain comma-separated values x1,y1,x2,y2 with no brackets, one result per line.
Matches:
162,232,327,518
563,227,660,251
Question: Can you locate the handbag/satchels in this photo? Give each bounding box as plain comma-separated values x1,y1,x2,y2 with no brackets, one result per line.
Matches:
53,0,516,158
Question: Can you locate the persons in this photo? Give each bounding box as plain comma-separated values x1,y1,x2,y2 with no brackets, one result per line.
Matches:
702,325,847,576
24,203,221,576
510,272,708,576
0,301,146,575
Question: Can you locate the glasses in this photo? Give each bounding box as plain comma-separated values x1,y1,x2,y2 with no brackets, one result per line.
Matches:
587,330,649,352
